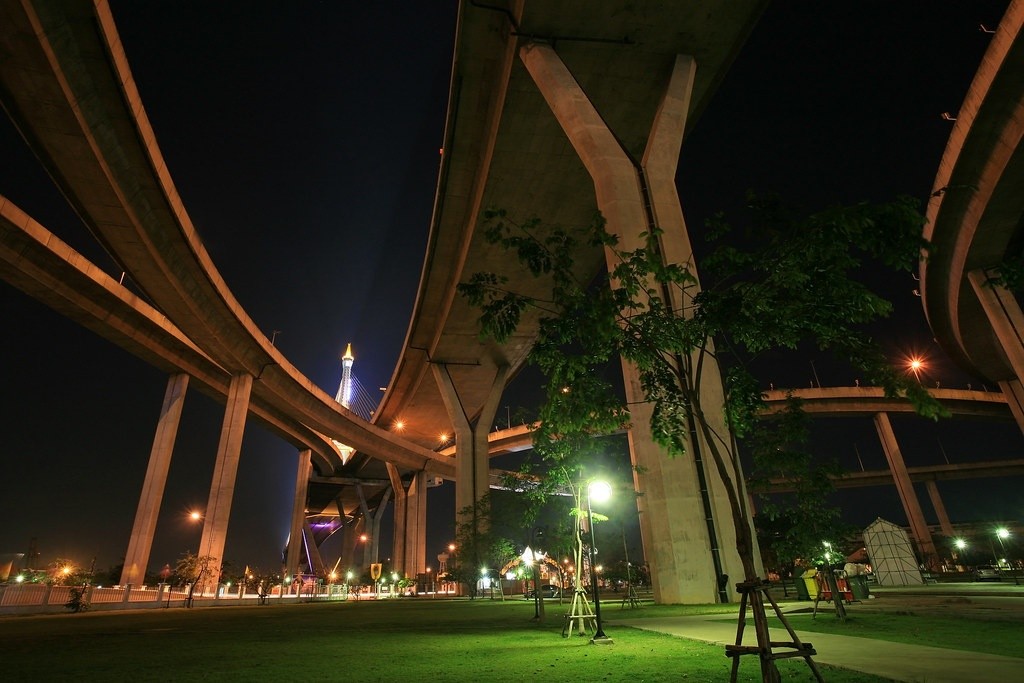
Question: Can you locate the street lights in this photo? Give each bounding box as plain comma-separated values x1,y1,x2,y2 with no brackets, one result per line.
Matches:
586,478,613,640
531,527,547,619
953,537,973,583
995,526,1021,586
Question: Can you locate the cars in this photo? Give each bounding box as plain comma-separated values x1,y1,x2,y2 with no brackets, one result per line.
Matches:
970,564,1001,582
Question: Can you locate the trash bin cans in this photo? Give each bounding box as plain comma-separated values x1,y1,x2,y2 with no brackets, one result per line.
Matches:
794,566,820,601
821,576,869,600
